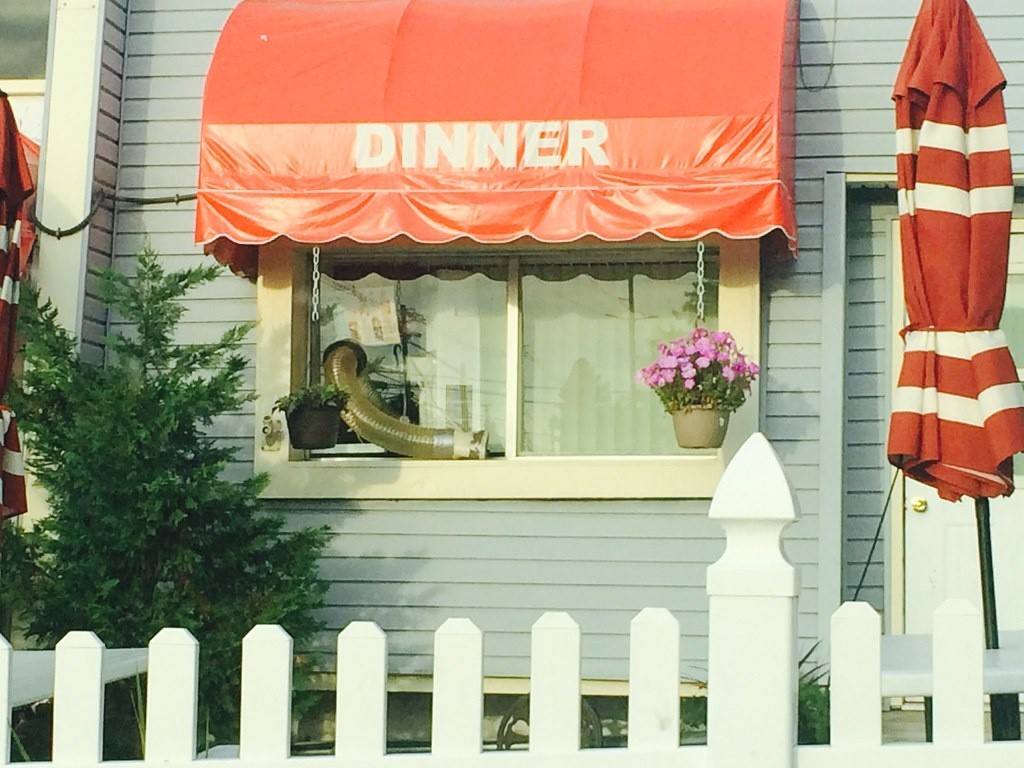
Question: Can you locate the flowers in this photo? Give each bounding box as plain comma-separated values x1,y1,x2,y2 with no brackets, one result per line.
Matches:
634,327,760,416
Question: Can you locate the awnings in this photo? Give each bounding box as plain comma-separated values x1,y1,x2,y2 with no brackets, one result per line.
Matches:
196,0,800,261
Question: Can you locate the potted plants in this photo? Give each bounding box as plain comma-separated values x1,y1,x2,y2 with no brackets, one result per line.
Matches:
272,384,351,450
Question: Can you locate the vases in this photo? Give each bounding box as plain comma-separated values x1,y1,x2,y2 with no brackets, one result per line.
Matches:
672,405,733,448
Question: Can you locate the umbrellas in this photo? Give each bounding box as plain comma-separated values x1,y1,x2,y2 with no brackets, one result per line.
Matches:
0,90,38,529
888,0,1024,651
